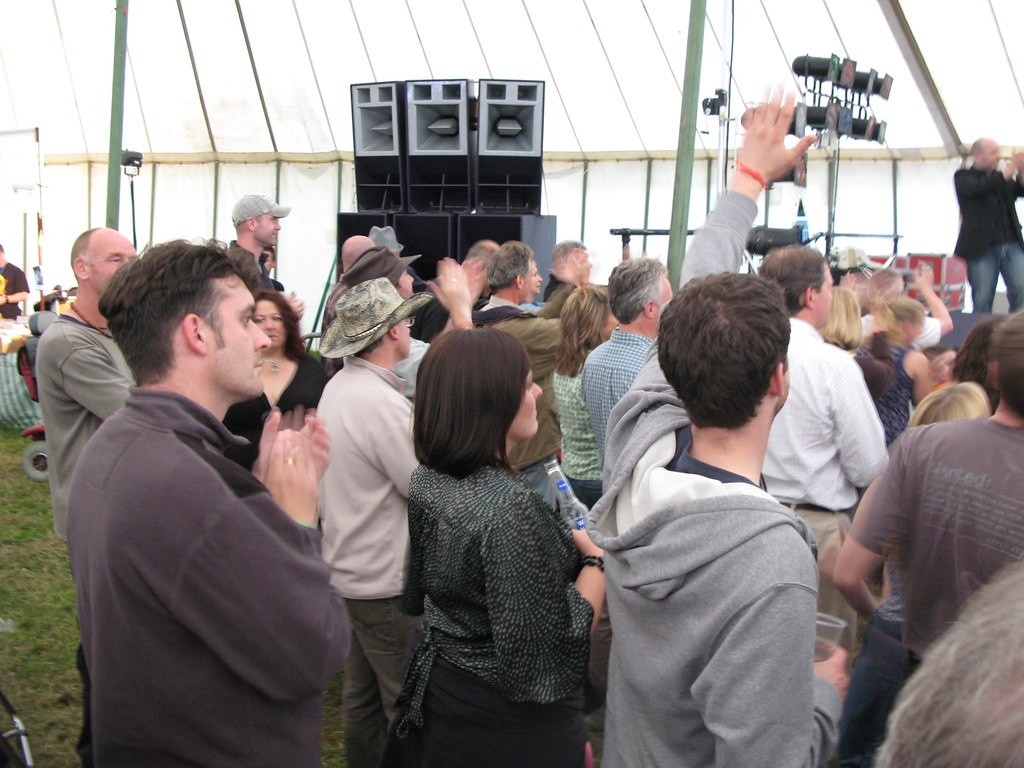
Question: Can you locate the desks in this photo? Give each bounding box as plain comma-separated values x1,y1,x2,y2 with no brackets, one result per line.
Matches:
0,316,42,431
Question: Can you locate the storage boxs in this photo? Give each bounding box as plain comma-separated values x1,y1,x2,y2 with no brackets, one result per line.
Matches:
874,253,968,313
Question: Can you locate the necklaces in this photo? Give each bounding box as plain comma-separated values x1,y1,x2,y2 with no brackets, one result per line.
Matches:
262,359,280,372
71,302,112,338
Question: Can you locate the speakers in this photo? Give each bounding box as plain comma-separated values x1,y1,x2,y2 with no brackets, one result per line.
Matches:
337,212,395,281
457,213,556,304
394,214,457,284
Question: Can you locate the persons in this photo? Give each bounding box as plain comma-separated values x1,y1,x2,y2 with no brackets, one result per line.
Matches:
552,283,619,512
874,560,1024,768
754,243,890,669
36,228,139,768
66,239,353,768
221,291,329,471
543,241,593,302
314,278,433,768
226,194,305,324
821,262,1024,768
321,225,500,402
473,241,593,511
0,244,30,320
954,138,1024,316
585,84,850,768
404,327,608,768
581,257,673,733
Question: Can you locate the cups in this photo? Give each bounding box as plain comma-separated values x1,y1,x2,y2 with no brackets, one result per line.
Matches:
814,611,849,662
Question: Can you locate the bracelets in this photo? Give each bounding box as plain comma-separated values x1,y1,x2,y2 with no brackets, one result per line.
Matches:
581,555,605,573
734,160,765,187
5,296,8,303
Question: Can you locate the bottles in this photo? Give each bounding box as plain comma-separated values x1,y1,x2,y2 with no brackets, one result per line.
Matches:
544,458,591,531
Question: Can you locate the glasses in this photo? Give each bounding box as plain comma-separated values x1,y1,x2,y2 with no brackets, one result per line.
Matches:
402,317,414,327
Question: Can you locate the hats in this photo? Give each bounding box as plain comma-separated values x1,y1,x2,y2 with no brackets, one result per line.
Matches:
369,226,404,253
232,194,291,228
319,277,434,359
342,246,423,285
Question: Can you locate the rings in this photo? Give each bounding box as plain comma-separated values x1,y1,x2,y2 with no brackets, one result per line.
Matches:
285,460,294,465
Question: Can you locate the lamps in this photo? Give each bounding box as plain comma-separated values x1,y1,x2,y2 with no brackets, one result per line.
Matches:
767,50,893,189
